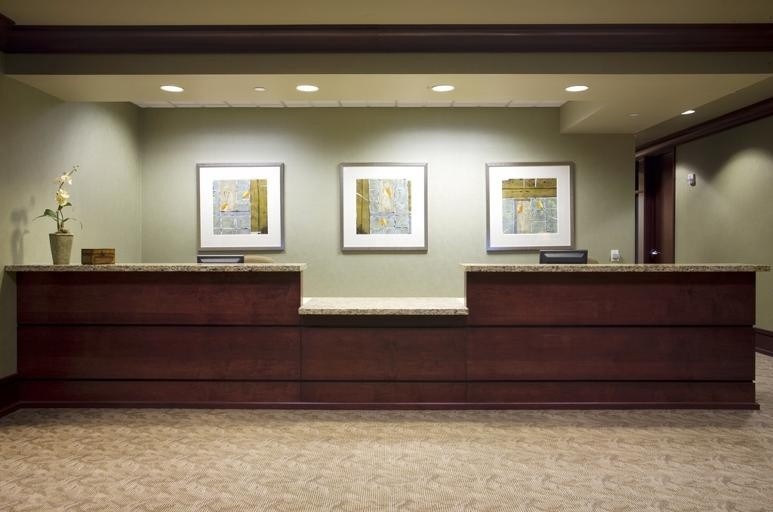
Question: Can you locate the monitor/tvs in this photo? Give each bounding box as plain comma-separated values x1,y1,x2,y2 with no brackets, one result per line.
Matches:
539,250,588,264
197,255,244,263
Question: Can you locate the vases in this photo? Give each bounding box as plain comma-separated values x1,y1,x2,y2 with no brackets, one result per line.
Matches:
48,233,74,266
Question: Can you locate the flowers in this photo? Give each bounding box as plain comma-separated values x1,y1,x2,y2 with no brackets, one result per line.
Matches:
31,163,84,232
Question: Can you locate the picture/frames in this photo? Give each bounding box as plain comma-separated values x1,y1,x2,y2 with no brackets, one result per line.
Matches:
338,161,429,252
196,161,285,252
484,161,578,252
